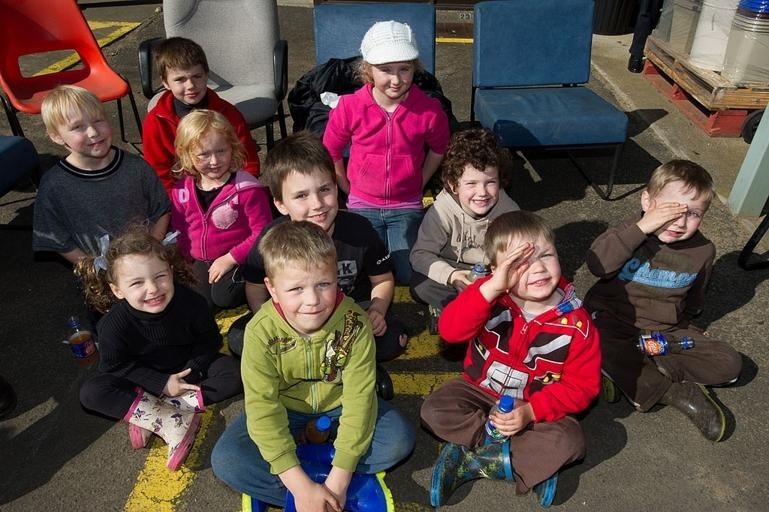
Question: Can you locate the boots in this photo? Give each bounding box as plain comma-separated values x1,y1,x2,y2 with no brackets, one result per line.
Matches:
599,367,623,403
240,442,395,512
484,435,559,508
658,381,727,441
125,383,204,471
430,440,513,506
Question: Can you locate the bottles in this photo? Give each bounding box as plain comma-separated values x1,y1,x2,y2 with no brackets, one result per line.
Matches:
467,263,487,283
306,415,331,442
482,394,515,446
634,333,695,355
69,316,99,364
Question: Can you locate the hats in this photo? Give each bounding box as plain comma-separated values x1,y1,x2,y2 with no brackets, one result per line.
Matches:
360,20,421,66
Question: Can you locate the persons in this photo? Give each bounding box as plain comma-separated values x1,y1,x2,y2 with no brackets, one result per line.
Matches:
626,0,667,75
78,223,243,472
421,210,602,507
209,220,415,512
32,85,174,334
410,124,527,364
167,108,275,310
323,20,451,285
583,160,742,442
140,37,264,209
227,130,407,403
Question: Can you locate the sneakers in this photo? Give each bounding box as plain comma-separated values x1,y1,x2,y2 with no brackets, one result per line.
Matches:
627,52,643,72
375,367,394,401
427,303,442,335
